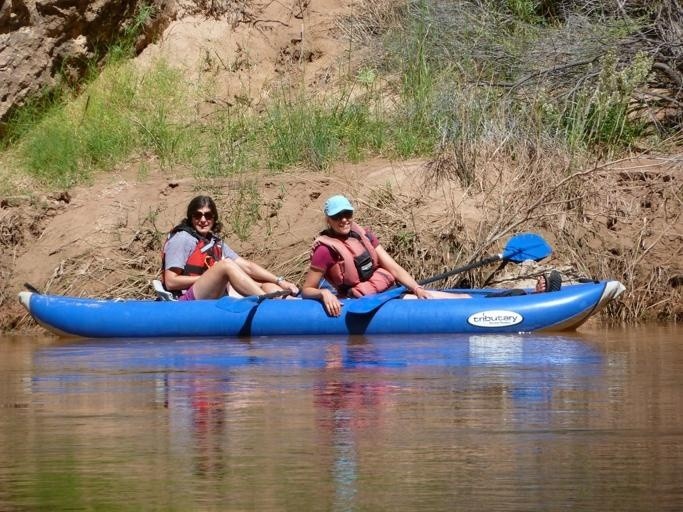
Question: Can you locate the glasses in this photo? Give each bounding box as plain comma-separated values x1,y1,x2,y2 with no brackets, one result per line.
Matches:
331,211,352,220
192,211,214,220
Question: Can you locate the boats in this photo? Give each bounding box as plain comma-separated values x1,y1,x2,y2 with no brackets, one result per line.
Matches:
30,338,601,396
15,277,626,339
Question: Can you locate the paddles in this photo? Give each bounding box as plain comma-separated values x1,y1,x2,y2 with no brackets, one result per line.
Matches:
216,290,293,312
344,233,552,315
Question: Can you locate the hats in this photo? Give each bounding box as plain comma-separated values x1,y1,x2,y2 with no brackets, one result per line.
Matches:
324,195,354,216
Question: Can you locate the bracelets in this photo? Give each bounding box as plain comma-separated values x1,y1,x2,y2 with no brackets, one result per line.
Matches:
414,285,424,295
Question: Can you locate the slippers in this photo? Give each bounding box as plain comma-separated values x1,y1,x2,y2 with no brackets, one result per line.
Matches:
540,270,562,291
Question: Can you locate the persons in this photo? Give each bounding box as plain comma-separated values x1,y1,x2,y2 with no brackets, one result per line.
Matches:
301,195,546,317
161,196,300,301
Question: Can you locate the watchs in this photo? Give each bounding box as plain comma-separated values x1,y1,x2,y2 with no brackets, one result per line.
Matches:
276,276,285,284
